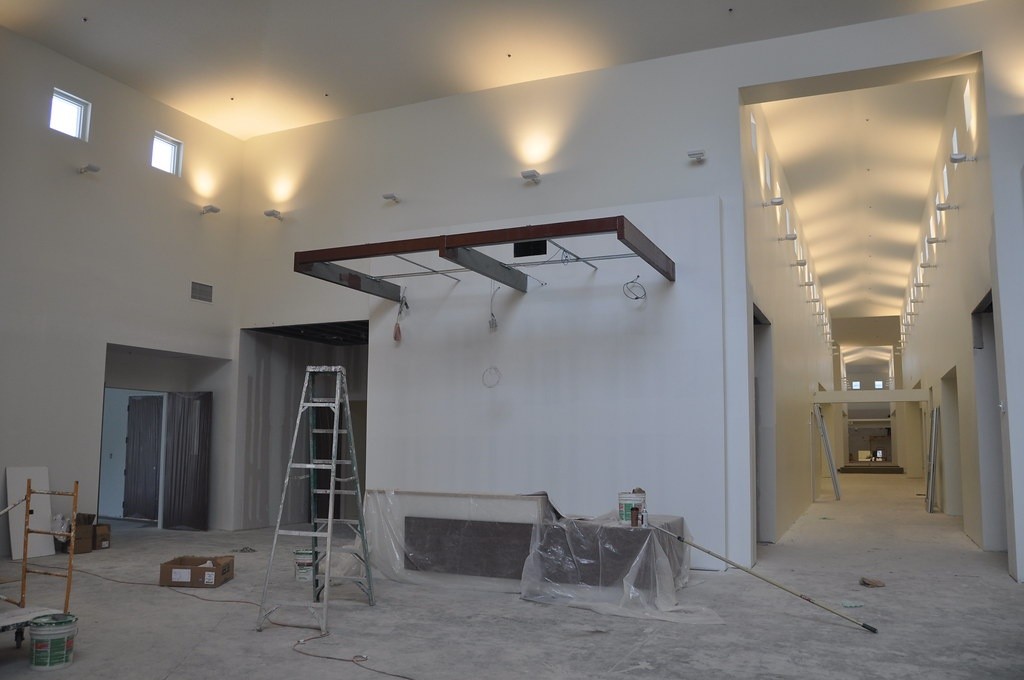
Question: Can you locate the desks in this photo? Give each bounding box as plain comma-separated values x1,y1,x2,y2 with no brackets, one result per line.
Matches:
572,515,683,589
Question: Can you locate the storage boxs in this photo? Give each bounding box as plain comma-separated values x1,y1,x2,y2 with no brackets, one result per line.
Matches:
160,556,235,589
63,513,96,554
93,523,111,549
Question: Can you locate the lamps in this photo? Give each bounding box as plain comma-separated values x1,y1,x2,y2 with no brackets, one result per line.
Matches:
926,238,946,244
895,282,928,356
791,260,807,267
202,205,220,214
80,163,99,174
519,170,541,183
948,154,976,162
800,281,838,356
263,210,284,223
383,193,400,204
763,197,784,207
686,150,705,162
920,263,937,268
779,234,797,240
936,203,958,211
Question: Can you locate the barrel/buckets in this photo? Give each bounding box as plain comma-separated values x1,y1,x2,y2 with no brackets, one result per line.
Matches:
619,492,646,524
292,549,320,581
29,613,79,671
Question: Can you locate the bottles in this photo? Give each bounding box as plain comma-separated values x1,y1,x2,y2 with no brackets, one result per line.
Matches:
642,509,648,527
631,507,639,526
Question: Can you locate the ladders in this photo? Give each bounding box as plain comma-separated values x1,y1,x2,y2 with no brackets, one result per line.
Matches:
255,364,377,635
